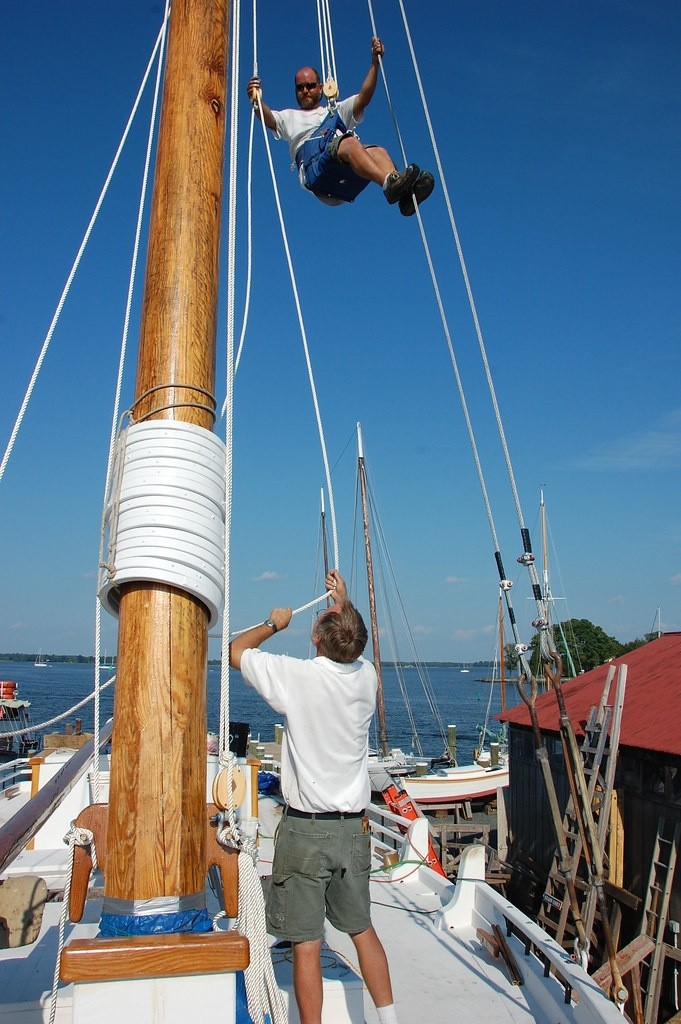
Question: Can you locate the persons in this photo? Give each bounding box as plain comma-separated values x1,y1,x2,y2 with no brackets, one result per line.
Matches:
246,37,435,216
230,571,399,1024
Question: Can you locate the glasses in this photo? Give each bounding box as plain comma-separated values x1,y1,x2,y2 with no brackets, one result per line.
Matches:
317,609,327,621
296,82,320,91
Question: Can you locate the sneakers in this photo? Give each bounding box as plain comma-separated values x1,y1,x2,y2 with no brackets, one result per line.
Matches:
398,171,435,217
383,163,420,205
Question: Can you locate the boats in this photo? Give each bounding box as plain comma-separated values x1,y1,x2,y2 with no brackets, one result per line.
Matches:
395,586,513,803
368,746,448,775
0,689,43,791
461,656,471,672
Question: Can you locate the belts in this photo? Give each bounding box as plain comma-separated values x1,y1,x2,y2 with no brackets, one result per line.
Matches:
282,805,366,820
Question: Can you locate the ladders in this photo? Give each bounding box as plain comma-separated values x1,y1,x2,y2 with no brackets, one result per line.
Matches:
636,815,681,1024
579,784,625,999
383,785,447,879
524,662,630,991
440,822,511,901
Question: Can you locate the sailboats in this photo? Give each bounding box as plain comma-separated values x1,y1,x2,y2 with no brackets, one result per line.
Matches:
34,646,47,666
99,647,116,669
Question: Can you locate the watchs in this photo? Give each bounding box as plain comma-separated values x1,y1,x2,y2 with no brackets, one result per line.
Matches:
264,619,277,633
